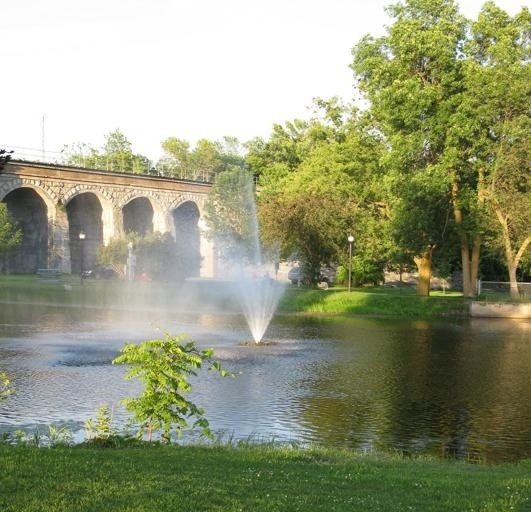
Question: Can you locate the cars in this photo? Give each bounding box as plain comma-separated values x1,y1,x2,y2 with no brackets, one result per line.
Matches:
82,263,118,280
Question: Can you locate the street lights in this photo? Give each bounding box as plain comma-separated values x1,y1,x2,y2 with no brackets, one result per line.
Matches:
347,233,355,290
79,229,86,284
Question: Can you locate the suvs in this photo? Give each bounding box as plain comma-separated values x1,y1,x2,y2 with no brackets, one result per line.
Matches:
288,266,329,285
